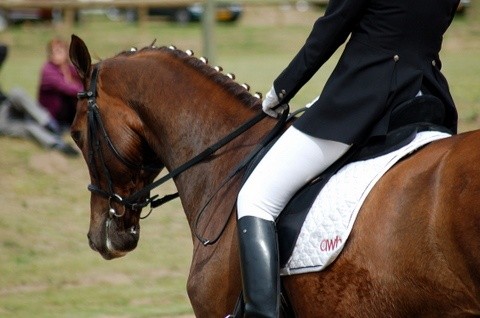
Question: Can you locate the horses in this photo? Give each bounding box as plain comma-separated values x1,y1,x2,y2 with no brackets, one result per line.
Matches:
68,33,480,318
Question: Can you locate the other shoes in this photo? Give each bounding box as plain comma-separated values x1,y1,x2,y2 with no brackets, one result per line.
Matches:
62,145,78,156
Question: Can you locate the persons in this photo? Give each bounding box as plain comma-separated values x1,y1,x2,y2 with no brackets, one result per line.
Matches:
38,38,89,135
237,0,461,318
0,45,79,156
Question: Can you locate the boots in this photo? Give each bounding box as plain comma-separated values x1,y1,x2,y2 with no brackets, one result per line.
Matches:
229,215,281,318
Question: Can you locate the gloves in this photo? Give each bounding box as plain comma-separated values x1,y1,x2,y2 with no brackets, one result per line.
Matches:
262,85,288,118
